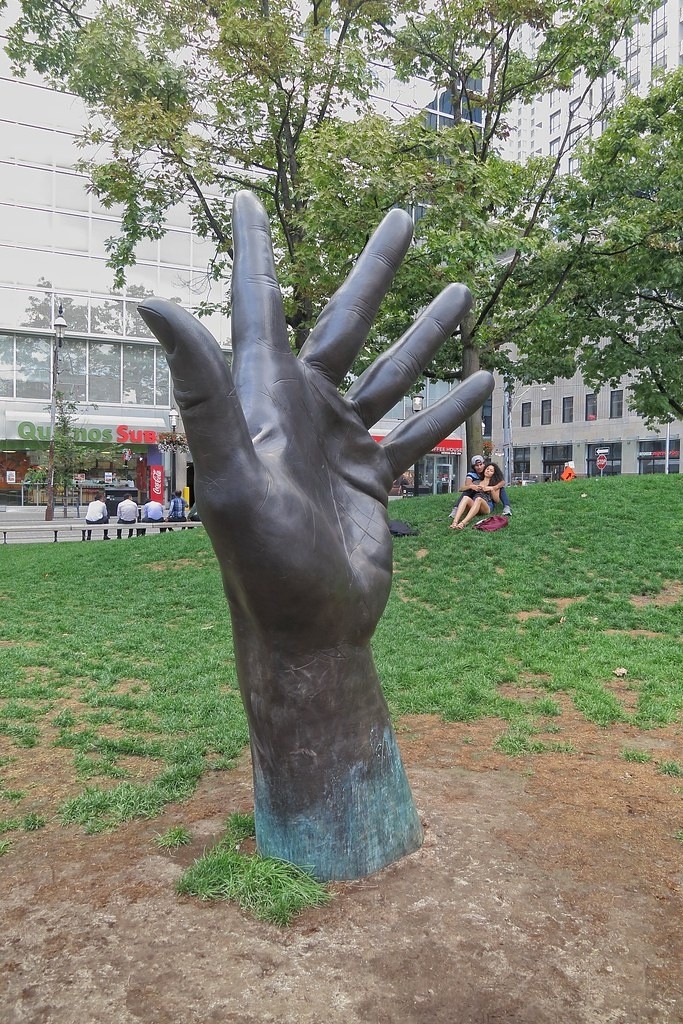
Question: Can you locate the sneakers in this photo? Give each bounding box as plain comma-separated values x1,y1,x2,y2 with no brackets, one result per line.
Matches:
502,506,512,516
449,507,457,518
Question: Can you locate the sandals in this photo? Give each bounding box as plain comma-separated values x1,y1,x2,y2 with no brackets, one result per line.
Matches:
449,520,457,528
456,521,466,530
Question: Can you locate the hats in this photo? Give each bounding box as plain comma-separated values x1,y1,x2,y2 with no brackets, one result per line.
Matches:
471,455,484,466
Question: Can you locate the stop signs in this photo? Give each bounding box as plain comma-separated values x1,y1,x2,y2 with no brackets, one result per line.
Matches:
597,454,607,470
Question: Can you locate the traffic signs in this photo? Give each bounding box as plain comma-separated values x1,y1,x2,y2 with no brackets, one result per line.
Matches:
594,447,610,456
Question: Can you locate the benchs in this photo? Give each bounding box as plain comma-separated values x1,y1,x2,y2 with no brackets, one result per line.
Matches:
0,520,202,545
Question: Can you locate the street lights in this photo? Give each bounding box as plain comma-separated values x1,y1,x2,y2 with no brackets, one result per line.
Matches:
169,403,179,500
410,390,425,497
504,384,548,487
44,303,68,522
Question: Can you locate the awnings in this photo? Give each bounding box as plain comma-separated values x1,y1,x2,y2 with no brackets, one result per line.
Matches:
367,429,463,455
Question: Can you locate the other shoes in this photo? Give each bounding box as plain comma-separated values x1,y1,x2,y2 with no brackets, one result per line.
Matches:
104,536,110,539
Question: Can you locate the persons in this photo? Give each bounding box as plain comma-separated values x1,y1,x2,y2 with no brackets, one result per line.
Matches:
85,493,111,540
140,499,166,535
125,476,135,500
398,475,409,499
116,493,139,539
137,189,497,878
450,462,503,529
110,472,117,499
165,489,188,531
448,455,512,518
187,502,201,529
23,468,38,503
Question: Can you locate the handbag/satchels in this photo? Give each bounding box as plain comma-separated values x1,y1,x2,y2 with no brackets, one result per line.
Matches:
472,515,508,533
